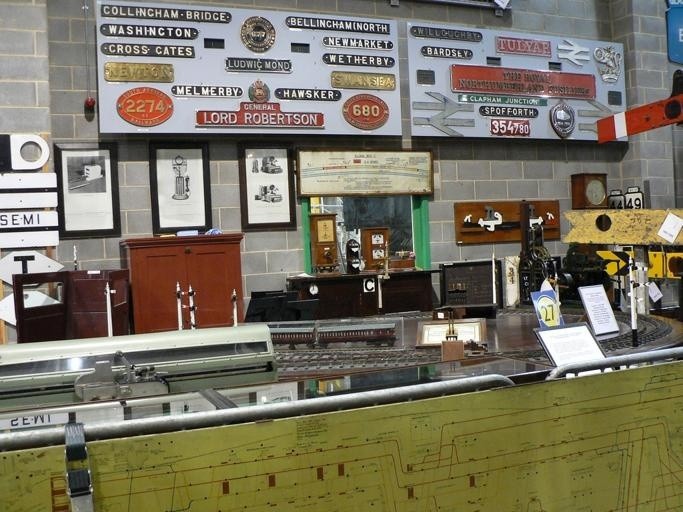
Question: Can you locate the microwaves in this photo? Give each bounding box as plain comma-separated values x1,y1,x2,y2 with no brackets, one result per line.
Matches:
439,256,519,307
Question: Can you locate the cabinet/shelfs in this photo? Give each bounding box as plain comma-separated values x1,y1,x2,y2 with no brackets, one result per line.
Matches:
286,269,444,319
120,232,245,334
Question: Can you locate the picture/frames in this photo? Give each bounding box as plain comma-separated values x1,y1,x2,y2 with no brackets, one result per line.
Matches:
238,140,297,232
149,140,213,236
53,141,121,240
